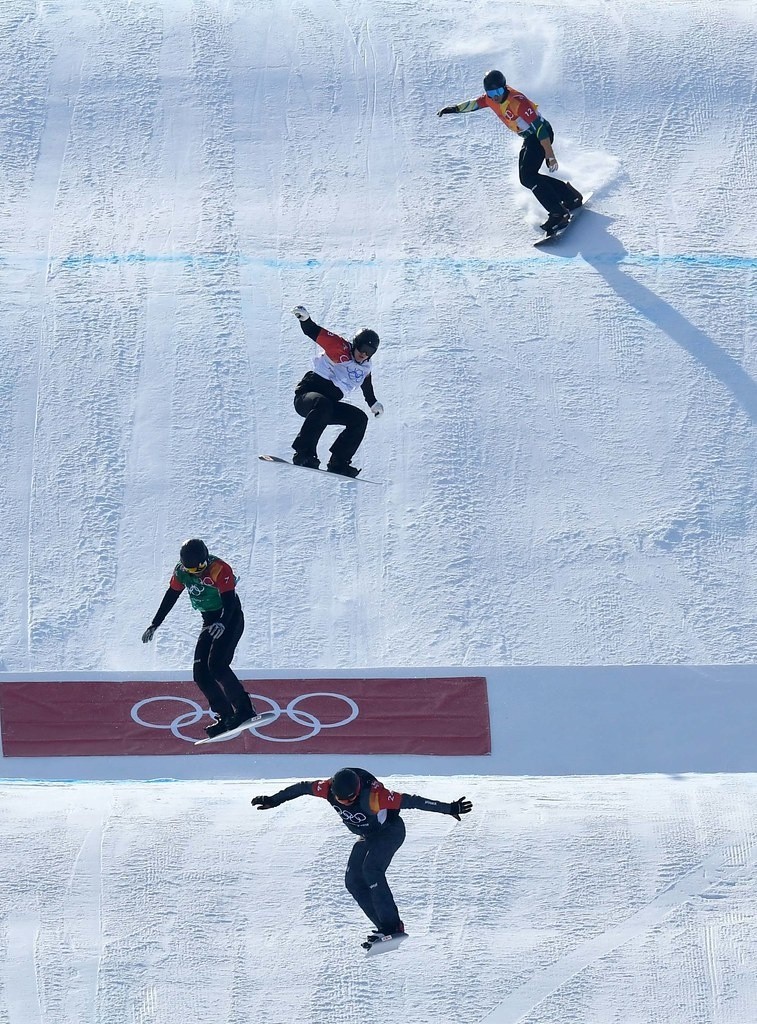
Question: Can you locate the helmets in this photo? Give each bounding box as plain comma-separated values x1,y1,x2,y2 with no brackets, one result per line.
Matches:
179,539,208,567
483,70,506,91
354,328,380,349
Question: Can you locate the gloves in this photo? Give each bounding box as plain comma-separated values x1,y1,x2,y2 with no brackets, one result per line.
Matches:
142,624,156,644
251,796,274,811
371,402,384,418
436,106,459,117
292,305,309,321
209,623,225,640
546,155,559,172
451,796,473,821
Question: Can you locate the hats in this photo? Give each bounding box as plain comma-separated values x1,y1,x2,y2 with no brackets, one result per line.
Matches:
331,769,359,798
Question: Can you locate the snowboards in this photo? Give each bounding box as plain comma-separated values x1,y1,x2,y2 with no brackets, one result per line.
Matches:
259,454,383,486
194,711,277,745
365,932,409,957
533,192,593,246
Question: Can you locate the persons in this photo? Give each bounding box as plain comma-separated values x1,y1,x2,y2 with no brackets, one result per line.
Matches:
437,69,583,234
251,767,474,950
291,306,384,478
142,538,256,737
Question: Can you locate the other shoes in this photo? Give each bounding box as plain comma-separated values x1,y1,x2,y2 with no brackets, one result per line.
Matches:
361,920,404,949
205,710,235,739
327,453,358,477
561,192,583,209
225,705,257,730
541,208,570,232
293,449,321,468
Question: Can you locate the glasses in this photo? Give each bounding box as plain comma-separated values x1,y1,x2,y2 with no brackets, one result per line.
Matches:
357,342,377,357
338,796,356,804
185,561,208,574
487,87,505,97
